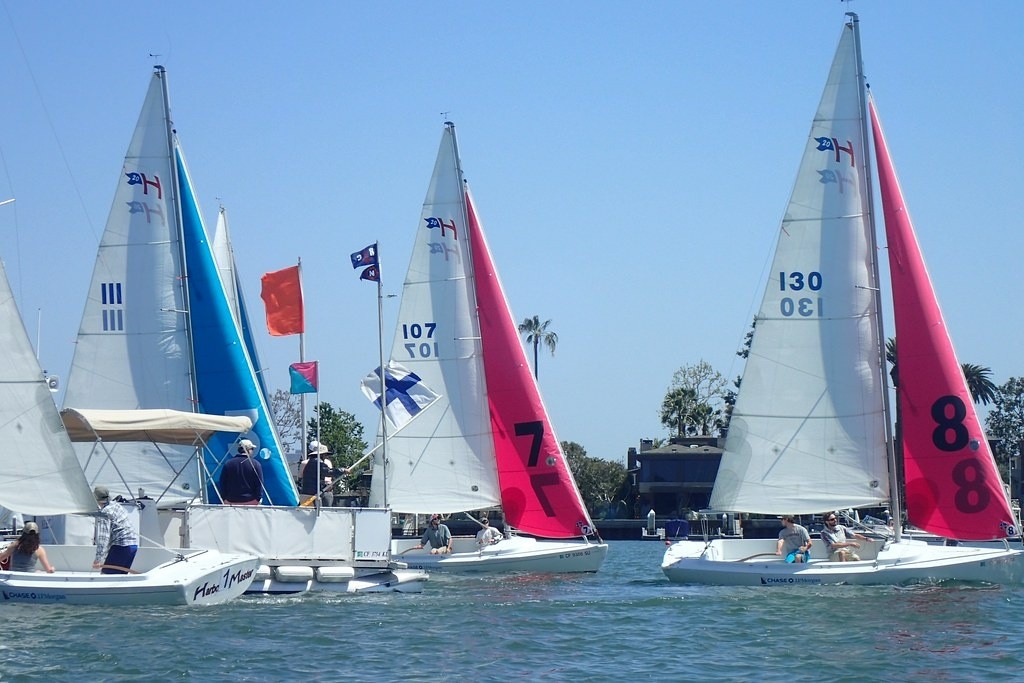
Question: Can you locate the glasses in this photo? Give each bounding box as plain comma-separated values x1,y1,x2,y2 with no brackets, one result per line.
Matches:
432,517,440,520
482,521,489,524
828,517,837,521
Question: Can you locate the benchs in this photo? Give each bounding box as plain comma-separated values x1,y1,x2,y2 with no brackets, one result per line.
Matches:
720,556,868,562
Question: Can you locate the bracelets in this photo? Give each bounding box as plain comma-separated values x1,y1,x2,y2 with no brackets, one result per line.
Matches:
866,537,869,541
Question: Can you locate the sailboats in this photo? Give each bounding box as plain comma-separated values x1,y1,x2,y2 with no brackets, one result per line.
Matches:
53,56,428,600
657,11,1023,592
365,120,611,581
1,238,264,609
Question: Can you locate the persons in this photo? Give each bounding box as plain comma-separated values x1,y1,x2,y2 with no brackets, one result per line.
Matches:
776,515,812,563
297,441,352,507
219,439,264,505
475,518,503,552
820,513,875,562
882,510,892,525
414,514,452,555
0,522,55,574
92,486,139,574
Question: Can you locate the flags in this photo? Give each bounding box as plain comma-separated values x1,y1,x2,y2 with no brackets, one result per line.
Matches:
261,266,304,336
350,243,378,269
360,263,380,283
360,360,439,429
289,361,319,394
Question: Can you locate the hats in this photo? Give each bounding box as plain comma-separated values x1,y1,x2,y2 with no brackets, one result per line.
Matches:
430,514,441,522
308,440,322,452
884,510,890,513
308,445,332,455
482,518,488,521
777,515,795,518
93,486,111,503
23,522,39,534
238,440,257,449
